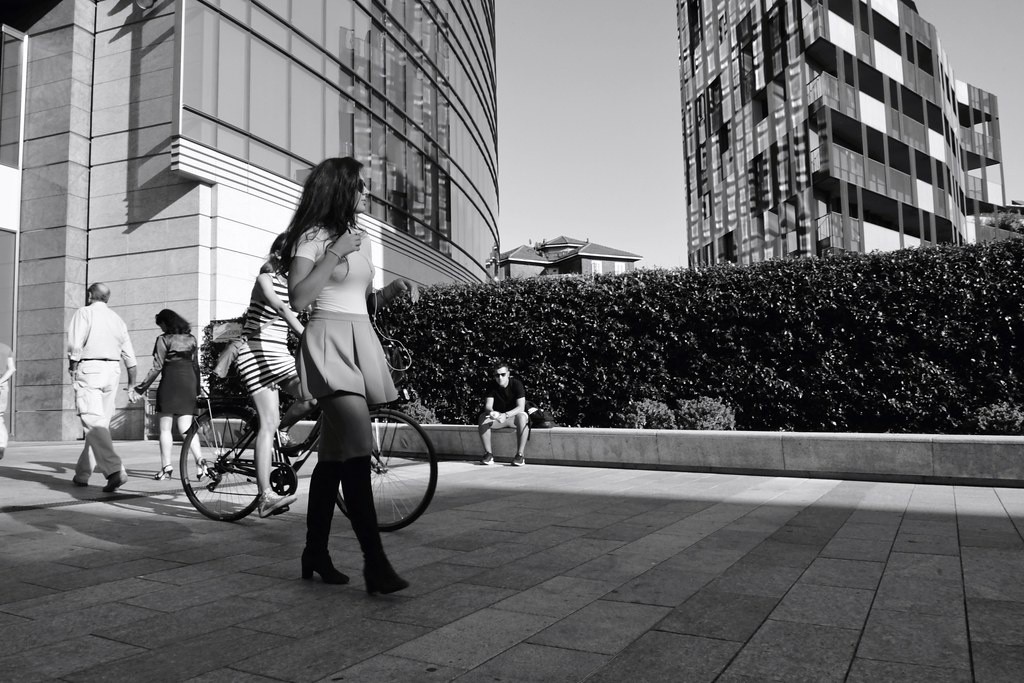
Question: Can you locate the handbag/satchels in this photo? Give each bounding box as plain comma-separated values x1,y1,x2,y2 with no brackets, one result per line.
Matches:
368,290,406,387
524,401,555,427
212,335,247,378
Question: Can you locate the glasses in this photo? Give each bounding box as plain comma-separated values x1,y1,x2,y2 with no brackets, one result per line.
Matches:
357,180,366,193
495,373,506,378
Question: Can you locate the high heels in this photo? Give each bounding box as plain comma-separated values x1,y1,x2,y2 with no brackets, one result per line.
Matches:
196,457,209,478
154,464,173,480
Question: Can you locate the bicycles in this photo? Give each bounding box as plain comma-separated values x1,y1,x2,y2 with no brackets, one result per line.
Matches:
179,340,438,532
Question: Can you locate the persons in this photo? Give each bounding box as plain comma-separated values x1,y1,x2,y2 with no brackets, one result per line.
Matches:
235,232,307,517
280,158,409,597
0,343,16,460
66,282,137,492
131,309,210,481
479,364,530,466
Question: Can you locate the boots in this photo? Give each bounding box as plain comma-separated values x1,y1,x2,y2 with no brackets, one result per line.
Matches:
341,455,409,596
301,460,349,584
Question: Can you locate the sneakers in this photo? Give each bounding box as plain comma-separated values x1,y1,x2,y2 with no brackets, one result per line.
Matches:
259,488,297,518
273,431,298,455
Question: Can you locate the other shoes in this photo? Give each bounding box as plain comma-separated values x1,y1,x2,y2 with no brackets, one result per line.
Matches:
103,470,128,491
511,454,525,466
480,452,494,465
72,475,87,486
